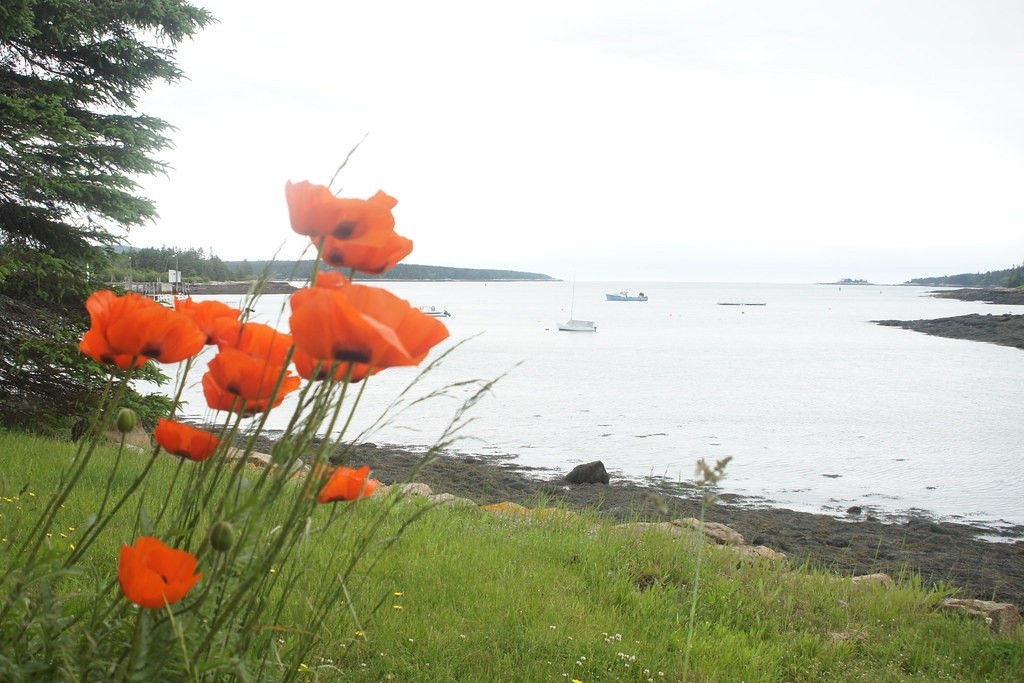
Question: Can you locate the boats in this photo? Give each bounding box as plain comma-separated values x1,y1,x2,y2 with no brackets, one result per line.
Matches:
149,294,188,308
605,291,648,302
717,288,766,306
419,305,452,318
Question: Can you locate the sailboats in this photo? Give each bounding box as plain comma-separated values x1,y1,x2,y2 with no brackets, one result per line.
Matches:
544,271,598,332
